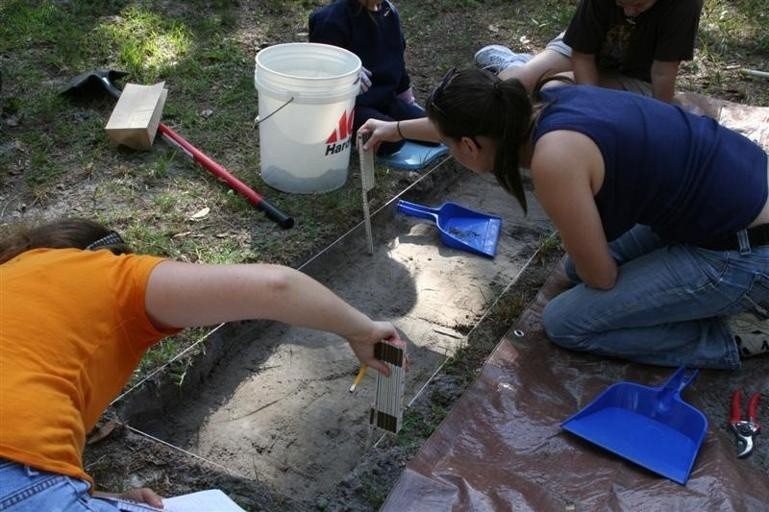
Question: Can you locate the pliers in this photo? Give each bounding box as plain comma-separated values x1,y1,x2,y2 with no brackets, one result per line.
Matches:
729,389,762,458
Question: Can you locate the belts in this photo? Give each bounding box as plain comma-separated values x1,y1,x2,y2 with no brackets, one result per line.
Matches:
697,222,769,253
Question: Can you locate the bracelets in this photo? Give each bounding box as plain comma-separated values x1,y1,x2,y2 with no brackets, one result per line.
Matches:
395,116,407,142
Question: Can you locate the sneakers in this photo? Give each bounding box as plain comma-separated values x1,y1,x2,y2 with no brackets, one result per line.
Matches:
724,311,769,357
473,44,535,74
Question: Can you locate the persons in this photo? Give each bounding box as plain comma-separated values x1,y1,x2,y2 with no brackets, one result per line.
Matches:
472,0,704,100
0,217,401,512
355,58,769,374
306,2,434,156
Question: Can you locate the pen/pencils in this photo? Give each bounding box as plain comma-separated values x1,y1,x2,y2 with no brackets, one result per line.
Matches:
350,363,368,393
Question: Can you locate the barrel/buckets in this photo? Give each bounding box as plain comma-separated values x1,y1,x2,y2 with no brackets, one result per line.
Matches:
253,42,363,194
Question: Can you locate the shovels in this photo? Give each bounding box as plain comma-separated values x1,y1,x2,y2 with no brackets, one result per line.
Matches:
58,70,294,229
